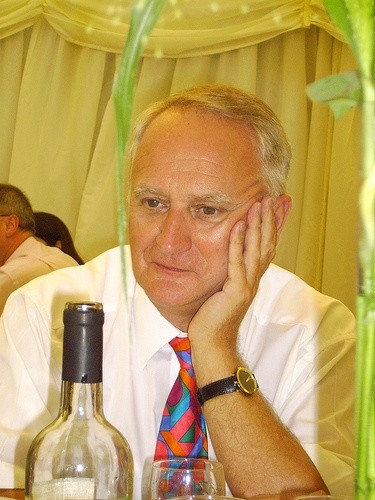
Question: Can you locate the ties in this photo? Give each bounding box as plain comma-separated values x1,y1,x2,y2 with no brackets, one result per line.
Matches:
153,337,208,500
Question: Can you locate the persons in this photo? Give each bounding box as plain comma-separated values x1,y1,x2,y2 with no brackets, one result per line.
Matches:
0,183,79,317
33,212,86,265
0,87,357,500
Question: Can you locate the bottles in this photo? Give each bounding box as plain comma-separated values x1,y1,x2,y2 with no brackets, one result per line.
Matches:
25,301,134,500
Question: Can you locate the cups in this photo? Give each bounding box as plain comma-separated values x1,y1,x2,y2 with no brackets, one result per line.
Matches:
149,458,246,499
295,494,349,500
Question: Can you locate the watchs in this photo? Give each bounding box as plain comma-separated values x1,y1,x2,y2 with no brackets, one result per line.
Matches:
197,367,259,405
